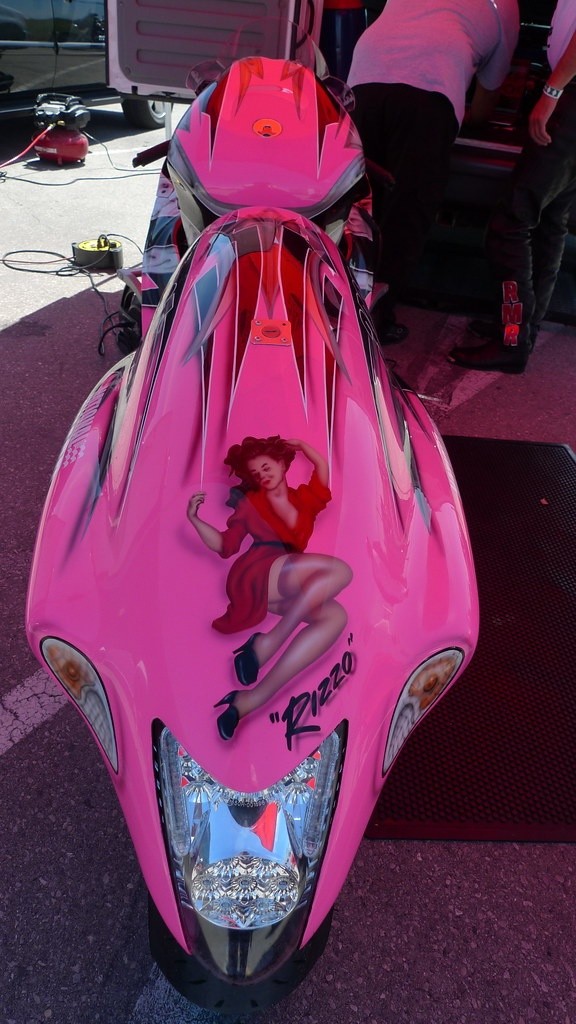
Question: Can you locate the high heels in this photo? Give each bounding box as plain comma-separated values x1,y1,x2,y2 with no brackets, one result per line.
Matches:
448,343,528,374
470,320,534,354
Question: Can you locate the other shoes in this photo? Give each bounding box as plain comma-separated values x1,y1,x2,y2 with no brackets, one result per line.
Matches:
378,322,409,343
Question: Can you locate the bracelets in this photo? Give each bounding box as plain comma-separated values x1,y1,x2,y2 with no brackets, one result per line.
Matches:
543,81,564,99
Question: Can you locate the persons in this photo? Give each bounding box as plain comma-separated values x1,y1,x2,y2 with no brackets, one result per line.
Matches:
445,0,576,374
343,0,520,348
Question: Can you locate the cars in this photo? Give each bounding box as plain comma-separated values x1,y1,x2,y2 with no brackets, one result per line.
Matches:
0,1,177,130
